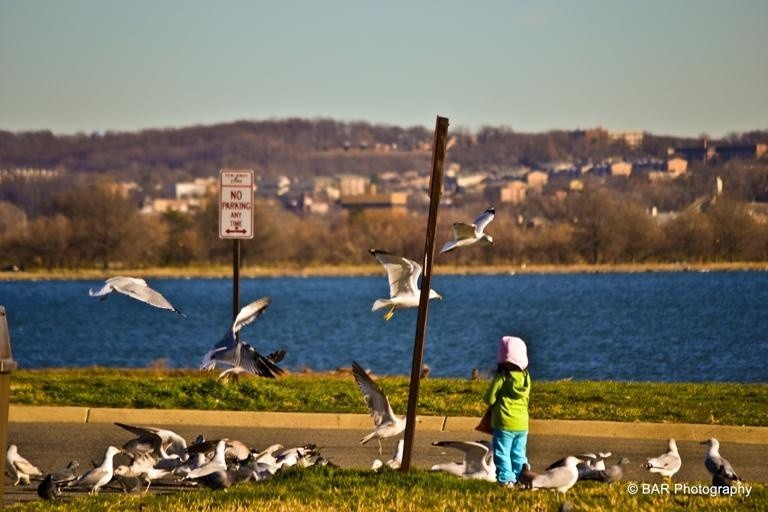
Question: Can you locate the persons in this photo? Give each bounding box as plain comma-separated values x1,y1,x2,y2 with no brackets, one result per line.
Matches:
483,334,532,487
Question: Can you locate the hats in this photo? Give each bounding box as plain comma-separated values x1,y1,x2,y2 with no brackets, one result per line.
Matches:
497,335,528,371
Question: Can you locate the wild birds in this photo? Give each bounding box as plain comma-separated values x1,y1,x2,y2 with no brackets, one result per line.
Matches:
37,445,122,501
431,438,496,483
87,275,188,321
646,437,683,483
519,451,632,500
114,422,340,494
439,205,496,255
385,439,404,471
368,246,442,320
6,444,43,486
700,436,743,483
198,295,287,382
351,360,421,456
